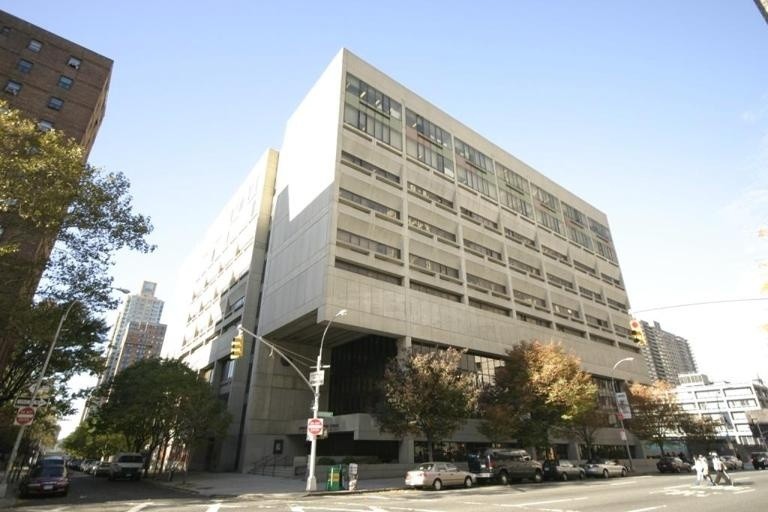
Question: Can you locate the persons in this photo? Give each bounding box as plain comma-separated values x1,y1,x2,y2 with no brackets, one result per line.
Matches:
691,452,734,487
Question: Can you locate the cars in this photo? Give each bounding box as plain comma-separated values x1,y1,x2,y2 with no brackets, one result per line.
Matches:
21,456,69,498
585,457,628,479
720,454,744,470
542,458,587,482
405,461,478,491
66,456,111,477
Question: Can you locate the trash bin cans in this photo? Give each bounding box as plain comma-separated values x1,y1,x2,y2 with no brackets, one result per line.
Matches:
328,466,339,490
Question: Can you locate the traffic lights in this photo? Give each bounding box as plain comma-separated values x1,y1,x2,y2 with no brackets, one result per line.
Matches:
229,335,244,361
629,319,647,347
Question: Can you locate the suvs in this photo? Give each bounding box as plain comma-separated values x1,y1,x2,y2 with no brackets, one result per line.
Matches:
656,456,692,473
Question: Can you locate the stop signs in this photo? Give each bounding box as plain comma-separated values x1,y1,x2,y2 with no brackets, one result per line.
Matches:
13,406,38,427
307,417,324,436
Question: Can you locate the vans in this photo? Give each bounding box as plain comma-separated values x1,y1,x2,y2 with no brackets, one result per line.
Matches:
111,452,144,481
471,444,545,485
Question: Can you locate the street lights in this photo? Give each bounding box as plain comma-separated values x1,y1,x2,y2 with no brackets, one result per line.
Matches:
305,309,348,494
0,286,132,498
611,357,636,472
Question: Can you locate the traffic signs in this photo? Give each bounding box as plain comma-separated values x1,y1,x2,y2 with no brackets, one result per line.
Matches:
13,396,50,410
